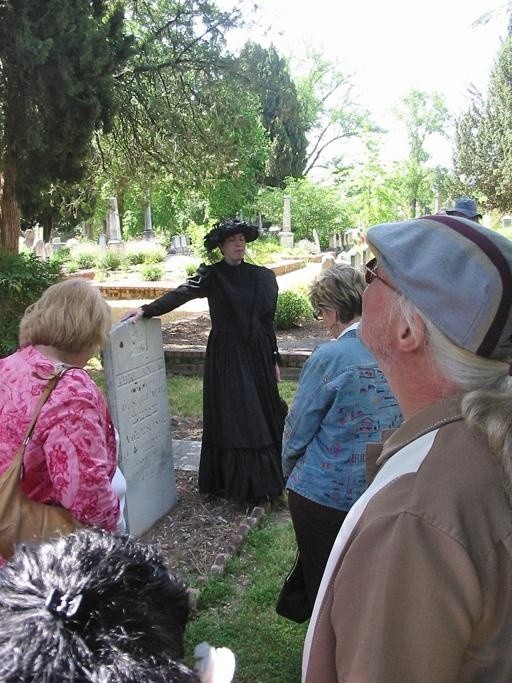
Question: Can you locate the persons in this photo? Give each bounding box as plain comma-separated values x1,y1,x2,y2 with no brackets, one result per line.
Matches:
296,208,511,682
445,197,481,223
278,261,399,622
119,218,297,514
1,271,131,573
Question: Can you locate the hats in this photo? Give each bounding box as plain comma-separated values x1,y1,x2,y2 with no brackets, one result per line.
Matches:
445,197,482,219
365,212,512,364
204,218,260,250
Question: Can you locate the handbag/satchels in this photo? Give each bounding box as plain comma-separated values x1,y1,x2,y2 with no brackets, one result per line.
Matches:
275,547,318,624
0,361,93,563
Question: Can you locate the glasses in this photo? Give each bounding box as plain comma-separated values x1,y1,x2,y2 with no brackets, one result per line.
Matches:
312,307,324,321
365,256,429,345
